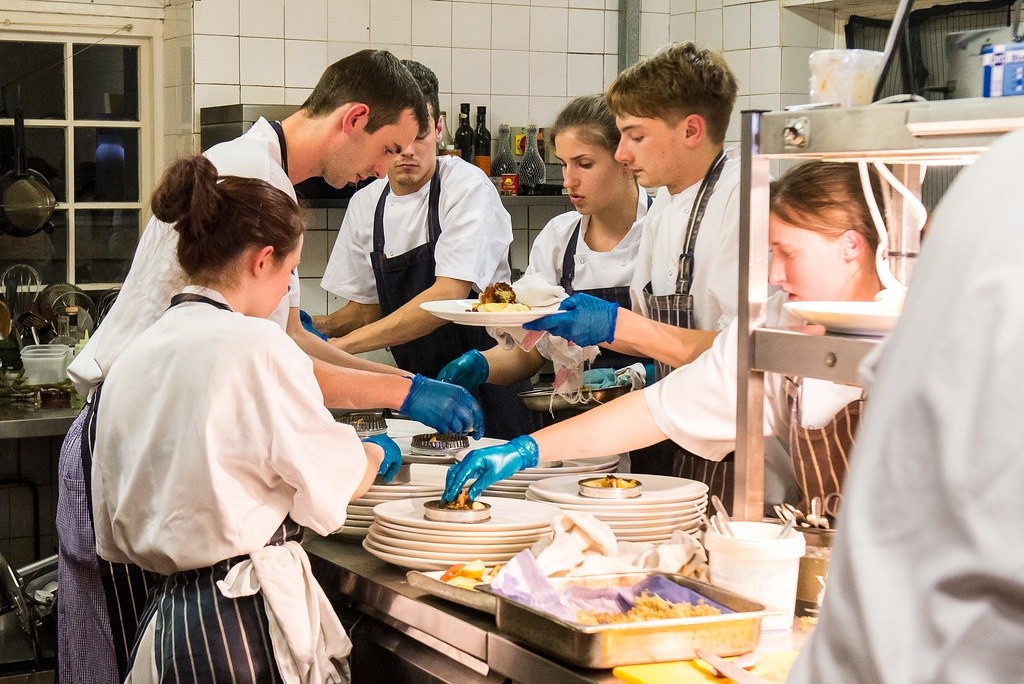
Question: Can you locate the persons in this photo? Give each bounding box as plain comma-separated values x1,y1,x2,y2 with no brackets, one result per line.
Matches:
785,119,1024,684
83,151,402,684
52,44,485,684
440,159,908,529
519,40,774,523
438,91,673,478
304,54,533,442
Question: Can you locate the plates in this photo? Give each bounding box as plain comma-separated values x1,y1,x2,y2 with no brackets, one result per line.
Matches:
420,299,567,326
783,301,897,336
342,419,709,571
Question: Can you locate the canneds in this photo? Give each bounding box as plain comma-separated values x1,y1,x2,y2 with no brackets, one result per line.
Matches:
502,173,519,196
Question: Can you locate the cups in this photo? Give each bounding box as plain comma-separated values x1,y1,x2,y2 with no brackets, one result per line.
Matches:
794,526,835,618
704,522,806,629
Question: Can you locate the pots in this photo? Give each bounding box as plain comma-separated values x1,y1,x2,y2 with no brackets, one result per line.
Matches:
0,108,59,237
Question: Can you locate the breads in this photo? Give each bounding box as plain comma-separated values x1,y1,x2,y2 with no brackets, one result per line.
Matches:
471,302,531,313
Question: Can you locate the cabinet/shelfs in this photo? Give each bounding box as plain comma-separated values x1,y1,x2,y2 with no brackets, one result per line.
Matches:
733,95,1024,517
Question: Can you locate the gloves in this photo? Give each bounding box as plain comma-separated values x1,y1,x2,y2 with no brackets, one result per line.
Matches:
362,434,403,482
300,308,327,340
523,292,620,347
440,434,540,505
400,373,484,441
436,349,489,391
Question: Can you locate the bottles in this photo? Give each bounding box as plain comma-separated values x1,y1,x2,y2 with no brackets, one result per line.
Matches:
455,103,474,164
75,329,90,354
518,125,546,195
439,111,453,149
491,125,518,177
473,106,491,176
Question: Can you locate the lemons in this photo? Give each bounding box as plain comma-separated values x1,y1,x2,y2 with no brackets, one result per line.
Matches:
460,559,485,578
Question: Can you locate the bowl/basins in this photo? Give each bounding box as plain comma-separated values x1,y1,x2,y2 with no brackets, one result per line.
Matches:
808,49,885,105
517,381,631,412
20,344,68,385
